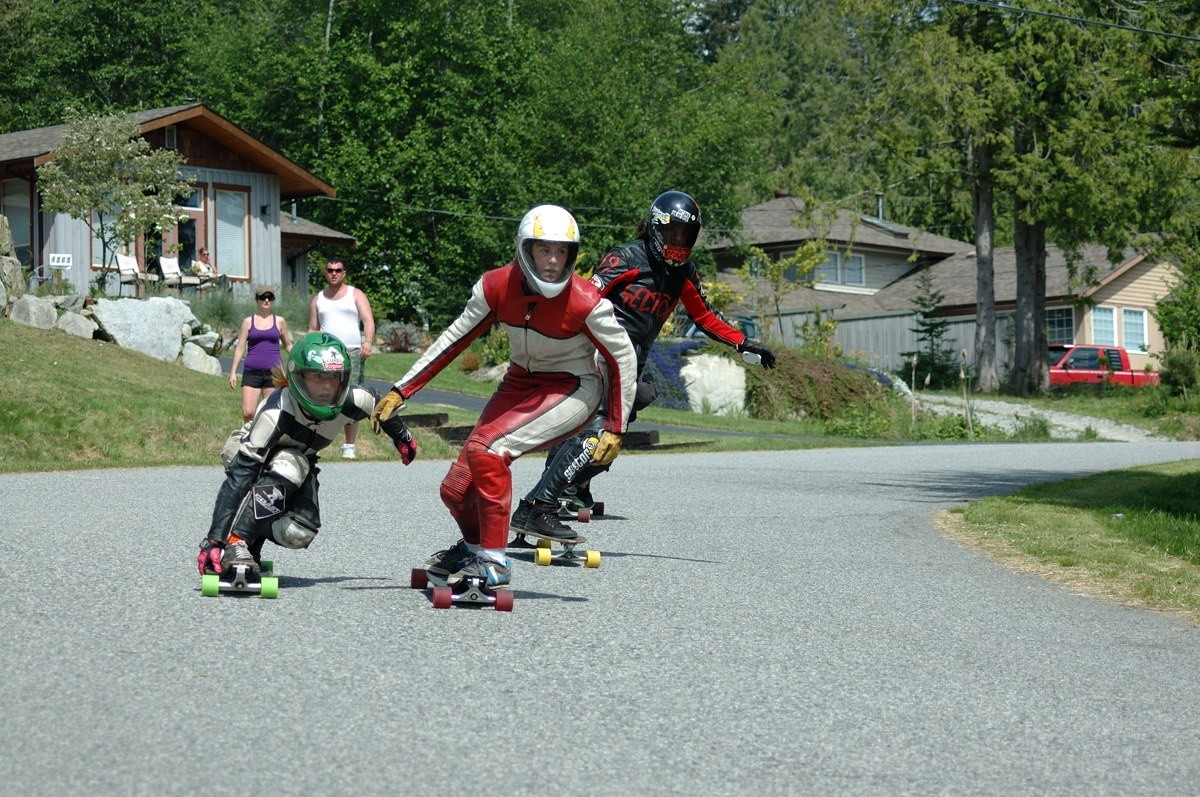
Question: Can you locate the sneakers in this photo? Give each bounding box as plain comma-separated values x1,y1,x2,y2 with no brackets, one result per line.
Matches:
507,498,571,530
446,557,511,587
221,540,261,573
422,536,476,575
524,505,578,539
247,548,271,577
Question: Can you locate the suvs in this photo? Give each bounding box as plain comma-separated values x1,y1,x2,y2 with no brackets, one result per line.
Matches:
673,312,761,346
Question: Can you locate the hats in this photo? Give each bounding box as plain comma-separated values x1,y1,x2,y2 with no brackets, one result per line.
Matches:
255,285,275,297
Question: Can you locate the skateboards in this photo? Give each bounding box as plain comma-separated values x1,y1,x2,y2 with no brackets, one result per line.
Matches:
508,525,601,568
556,498,605,524
201,559,279,600
410,559,514,612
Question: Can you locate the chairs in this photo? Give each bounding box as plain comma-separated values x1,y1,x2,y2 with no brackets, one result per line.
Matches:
156,254,203,299
115,254,160,298
190,260,252,295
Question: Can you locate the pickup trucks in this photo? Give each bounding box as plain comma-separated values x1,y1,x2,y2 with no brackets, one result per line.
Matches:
1044,344,1160,386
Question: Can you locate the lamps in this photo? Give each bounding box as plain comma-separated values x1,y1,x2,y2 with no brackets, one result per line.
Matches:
261,205,272,215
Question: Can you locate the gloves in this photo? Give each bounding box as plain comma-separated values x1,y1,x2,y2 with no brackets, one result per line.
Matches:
588,432,623,466
393,425,417,465
740,338,778,371
197,539,222,575
370,390,404,435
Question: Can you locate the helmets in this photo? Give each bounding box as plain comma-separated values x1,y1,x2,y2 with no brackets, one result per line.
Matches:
650,191,701,272
286,332,351,419
517,204,580,297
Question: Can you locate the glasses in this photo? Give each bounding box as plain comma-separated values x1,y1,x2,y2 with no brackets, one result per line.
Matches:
257,294,274,301
326,268,343,274
201,253,208,256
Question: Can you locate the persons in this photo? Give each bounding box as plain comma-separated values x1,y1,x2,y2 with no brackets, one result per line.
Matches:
371,206,637,589
229,285,293,423
194,247,233,291
197,331,417,576
308,259,375,459
508,191,775,539
